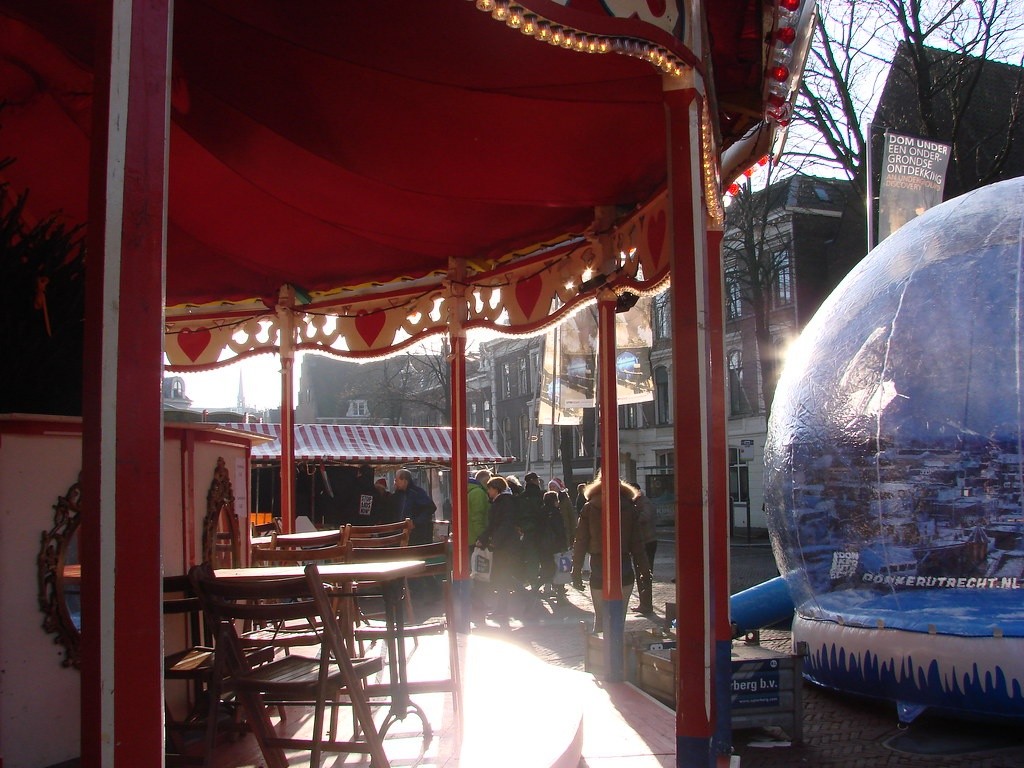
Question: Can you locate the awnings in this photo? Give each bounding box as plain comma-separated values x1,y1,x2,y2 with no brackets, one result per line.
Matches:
194,422,516,465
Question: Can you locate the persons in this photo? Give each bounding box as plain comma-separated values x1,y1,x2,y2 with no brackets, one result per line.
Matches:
468,469,578,616
393,470,442,601
631,484,656,611
375,479,391,495
573,469,652,632
340,465,383,526
576,483,592,581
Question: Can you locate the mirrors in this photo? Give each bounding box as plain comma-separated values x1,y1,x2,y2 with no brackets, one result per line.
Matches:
198,456,240,629
36,469,85,673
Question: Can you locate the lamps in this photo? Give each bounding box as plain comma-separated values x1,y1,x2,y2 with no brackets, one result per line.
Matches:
578,274,640,314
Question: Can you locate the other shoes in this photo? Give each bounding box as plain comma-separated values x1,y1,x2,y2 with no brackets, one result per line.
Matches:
488,608,508,617
632,605,652,613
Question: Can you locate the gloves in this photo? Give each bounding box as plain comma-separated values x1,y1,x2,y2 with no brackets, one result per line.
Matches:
572,568,586,591
637,562,652,584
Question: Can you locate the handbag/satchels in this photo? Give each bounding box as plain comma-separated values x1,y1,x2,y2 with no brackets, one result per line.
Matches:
559,554,571,572
470,546,493,583
553,550,573,585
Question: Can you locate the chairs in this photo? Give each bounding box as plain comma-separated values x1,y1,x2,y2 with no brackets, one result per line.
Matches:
161,518,463,768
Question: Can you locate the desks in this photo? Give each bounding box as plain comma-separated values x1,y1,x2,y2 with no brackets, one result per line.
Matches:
213,559,428,723
63,563,81,587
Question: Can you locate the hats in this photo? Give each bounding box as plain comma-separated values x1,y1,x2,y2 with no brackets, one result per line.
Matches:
524,472,540,482
552,477,569,492
375,478,390,493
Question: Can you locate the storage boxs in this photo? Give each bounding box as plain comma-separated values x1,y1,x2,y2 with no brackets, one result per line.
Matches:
578,619,809,745
